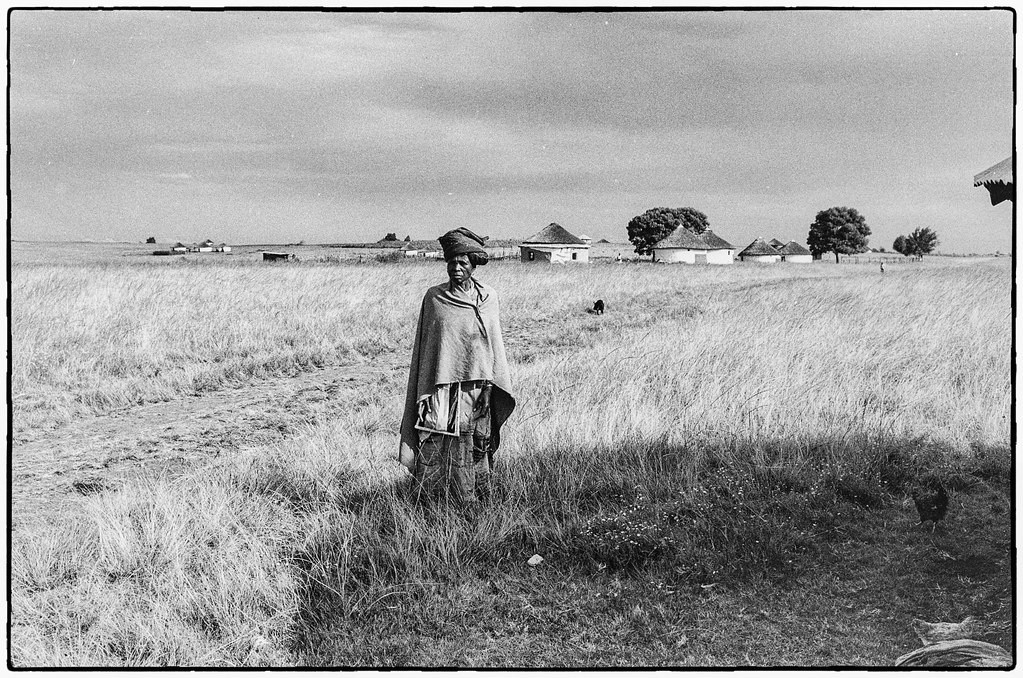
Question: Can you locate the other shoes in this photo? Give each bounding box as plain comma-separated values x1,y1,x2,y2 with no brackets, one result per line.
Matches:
463,502,479,524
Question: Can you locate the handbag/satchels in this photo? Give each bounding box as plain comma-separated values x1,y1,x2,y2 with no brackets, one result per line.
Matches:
415,372,466,441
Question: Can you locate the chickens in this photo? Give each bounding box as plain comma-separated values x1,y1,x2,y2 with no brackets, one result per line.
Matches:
911,485,950,533
910,617,985,646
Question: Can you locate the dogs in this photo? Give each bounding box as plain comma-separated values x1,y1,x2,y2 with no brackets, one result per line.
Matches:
593,300,604,315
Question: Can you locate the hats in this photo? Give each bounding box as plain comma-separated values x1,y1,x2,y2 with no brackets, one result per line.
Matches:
437,226,491,270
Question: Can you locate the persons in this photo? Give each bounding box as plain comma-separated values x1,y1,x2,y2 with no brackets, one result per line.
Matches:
397,244,517,508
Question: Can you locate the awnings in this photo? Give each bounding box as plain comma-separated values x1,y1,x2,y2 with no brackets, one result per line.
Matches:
972,153,1013,206
